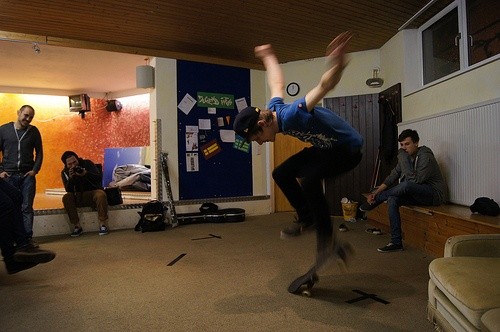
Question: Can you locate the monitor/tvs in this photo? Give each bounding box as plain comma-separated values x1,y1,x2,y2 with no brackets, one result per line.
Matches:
68,94,90,112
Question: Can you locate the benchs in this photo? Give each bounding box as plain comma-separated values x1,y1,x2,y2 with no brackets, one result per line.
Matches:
366,201,500,257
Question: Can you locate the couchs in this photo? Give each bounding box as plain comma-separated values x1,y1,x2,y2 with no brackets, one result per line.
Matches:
427,234,500,332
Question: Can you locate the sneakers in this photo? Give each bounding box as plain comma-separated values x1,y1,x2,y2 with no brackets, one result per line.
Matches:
71,225,83,236
98,224,108,236
377,242,403,252
359,201,377,212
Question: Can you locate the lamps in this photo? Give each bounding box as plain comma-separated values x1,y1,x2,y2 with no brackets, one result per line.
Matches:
32,43,40,54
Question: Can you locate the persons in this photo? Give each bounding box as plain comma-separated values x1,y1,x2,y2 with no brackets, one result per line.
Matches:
358,128,445,253
0,178,56,275
61,150,109,236
233,31,364,256
0,105,43,247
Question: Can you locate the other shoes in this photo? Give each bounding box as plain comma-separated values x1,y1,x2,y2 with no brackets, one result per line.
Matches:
20,241,39,250
280,215,312,238
14,250,56,264
6,260,40,275
366,228,381,234
338,223,347,231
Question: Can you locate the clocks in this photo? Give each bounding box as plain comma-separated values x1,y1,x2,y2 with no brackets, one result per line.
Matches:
286,82,301,96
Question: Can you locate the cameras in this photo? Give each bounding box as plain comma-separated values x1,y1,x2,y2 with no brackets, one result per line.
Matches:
72,167,84,174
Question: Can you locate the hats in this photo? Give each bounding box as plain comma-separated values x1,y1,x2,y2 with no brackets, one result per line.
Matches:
232,106,261,147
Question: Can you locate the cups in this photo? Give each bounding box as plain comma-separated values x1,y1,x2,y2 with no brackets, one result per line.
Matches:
341,197,350,204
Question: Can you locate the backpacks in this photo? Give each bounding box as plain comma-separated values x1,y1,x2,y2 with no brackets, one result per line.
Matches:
135,200,169,232
